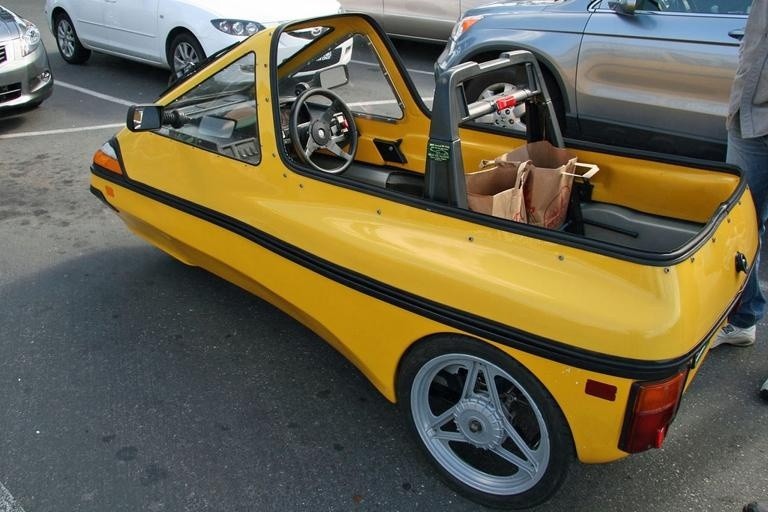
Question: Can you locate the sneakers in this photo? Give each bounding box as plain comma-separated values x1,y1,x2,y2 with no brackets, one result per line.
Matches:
703,322,756,349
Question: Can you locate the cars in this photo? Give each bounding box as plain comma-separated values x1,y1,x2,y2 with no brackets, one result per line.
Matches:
1,6,54,117
44,2,354,93
335,0,509,52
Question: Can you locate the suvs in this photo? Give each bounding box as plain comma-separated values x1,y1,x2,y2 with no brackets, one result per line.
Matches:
432,0,755,151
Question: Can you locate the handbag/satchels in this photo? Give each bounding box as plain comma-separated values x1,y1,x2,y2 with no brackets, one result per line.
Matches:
499,139,599,228
460,160,531,226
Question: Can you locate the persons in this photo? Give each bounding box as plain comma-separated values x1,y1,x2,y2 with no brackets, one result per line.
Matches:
708,0,768,395
